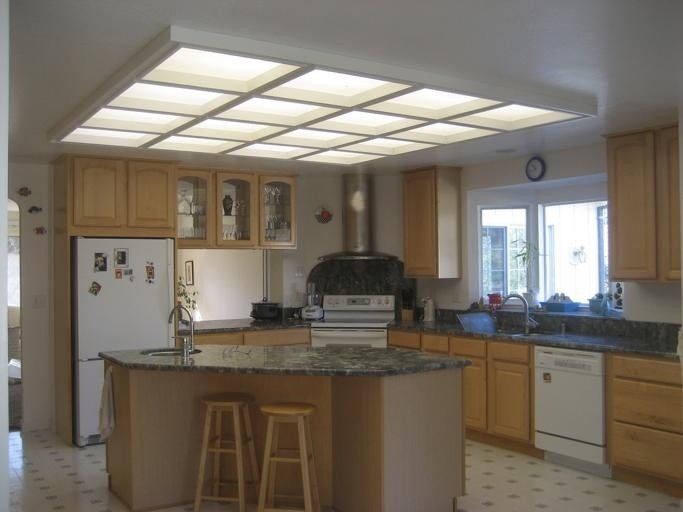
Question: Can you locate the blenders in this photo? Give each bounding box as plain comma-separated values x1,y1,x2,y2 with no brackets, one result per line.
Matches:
300,282,325,320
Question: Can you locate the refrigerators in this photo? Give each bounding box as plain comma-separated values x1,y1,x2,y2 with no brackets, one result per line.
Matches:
72,235,179,448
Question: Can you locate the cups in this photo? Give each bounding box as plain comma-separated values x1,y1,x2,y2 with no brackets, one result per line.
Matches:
264,183,280,205
266,214,291,241
223,225,248,240
178,224,206,241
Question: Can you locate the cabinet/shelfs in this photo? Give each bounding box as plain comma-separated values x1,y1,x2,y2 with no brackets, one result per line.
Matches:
492,342,531,457
599,121,683,285
175,168,213,249
609,356,681,500
48,153,182,238
216,170,300,252
401,164,462,281
422,337,488,445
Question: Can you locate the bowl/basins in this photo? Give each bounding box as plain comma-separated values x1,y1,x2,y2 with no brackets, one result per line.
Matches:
540,301,580,312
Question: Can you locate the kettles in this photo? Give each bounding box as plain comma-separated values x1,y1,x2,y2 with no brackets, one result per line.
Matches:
421,295,436,321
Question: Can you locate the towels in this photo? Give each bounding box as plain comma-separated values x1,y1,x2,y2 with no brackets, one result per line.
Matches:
96,370,117,444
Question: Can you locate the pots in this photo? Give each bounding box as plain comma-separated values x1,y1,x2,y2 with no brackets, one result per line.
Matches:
250,297,280,321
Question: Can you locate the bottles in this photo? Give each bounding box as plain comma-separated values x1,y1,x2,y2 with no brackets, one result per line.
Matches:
488,292,503,332
191,198,199,214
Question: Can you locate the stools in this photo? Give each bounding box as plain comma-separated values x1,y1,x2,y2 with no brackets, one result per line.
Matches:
193,391,322,512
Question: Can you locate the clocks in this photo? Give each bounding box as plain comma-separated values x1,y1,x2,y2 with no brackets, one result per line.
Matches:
525,157,547,181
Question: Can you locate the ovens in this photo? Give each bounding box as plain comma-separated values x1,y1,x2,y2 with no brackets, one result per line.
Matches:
310,328,387,349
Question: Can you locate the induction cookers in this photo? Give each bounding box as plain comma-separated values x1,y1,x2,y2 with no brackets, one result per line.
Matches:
312,313,394,331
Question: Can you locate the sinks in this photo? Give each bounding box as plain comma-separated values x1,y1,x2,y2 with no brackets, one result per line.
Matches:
512,333,542,337
140,348,202,356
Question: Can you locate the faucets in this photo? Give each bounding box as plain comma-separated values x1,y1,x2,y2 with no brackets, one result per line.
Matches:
168,305,194,351
501,294,528,335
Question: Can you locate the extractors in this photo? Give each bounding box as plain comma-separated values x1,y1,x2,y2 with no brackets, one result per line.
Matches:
321,169,397,264
